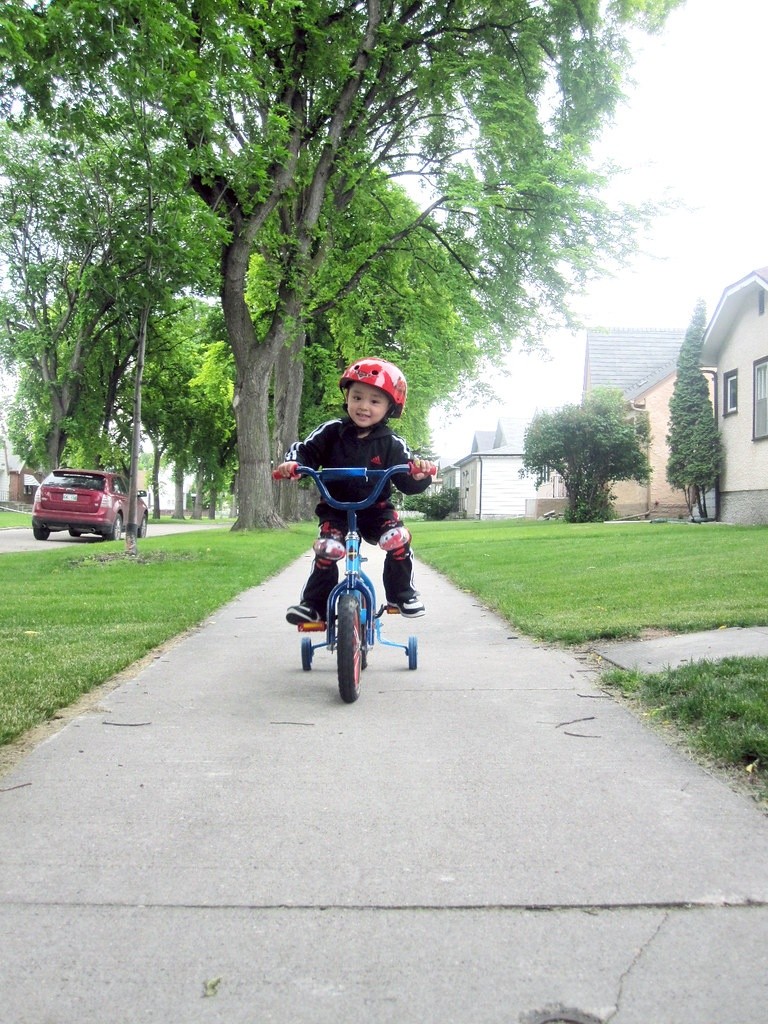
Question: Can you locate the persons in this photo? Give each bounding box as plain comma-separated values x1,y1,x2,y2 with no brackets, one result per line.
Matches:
286,358,436,626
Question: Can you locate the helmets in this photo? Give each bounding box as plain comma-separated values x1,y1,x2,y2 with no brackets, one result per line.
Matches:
340,358,408,418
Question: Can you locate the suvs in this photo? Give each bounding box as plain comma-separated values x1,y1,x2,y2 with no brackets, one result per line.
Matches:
32,465,149,543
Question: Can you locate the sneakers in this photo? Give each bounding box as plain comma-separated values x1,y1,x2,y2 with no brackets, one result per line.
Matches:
285,600,323,625
387,597,426,618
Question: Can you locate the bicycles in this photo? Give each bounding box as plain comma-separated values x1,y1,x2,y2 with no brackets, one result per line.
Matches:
272,461,438,706
